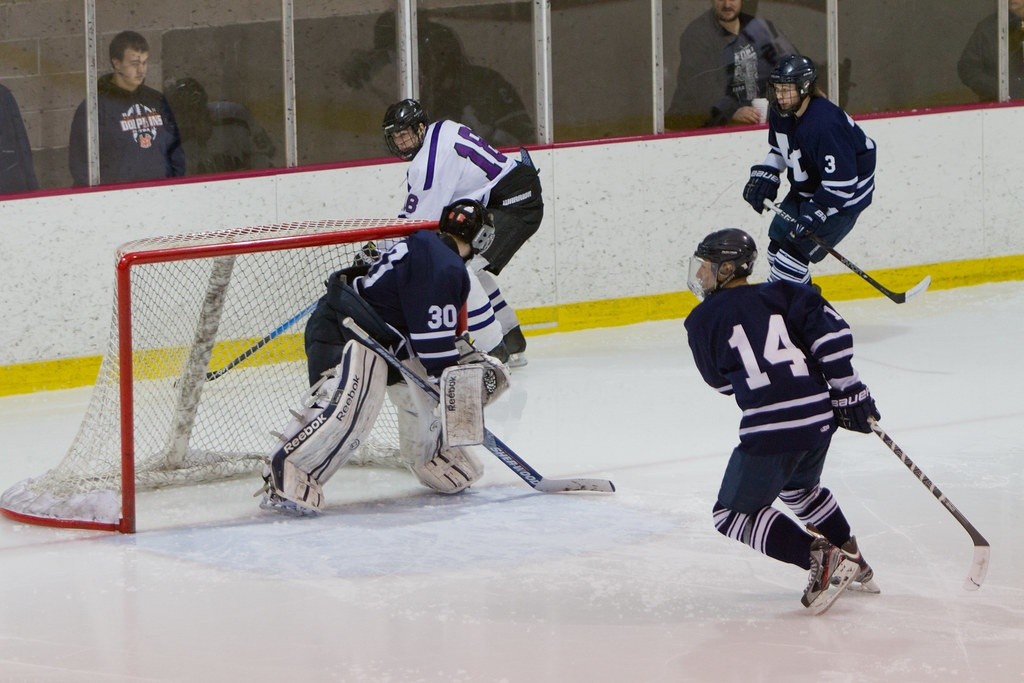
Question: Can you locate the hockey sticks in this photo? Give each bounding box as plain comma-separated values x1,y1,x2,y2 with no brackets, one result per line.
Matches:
763,197,932,305
342,316,616,493
173,300,320,389
865,413,991,594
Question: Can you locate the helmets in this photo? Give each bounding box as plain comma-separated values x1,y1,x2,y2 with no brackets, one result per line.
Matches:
768,54,817,118
382,99,429,162
694,228,758,282
439,198,495,261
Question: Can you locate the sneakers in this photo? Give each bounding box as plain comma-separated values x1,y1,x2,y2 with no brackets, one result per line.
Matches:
503,325,528,368
801,537,861,617
487,339,510,372
841,535,881,594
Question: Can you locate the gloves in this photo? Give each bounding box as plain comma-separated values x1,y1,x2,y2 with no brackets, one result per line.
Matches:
743,165,781,214
784,200,828,242
829,384,881,434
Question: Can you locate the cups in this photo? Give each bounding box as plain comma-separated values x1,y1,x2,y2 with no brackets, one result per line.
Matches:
751,97,770,123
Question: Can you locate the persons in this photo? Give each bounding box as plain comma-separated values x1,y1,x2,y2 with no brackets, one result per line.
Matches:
381,97,545,375
667,0,796,126
351,10,538,146
0,85,37,192
683,227,882,617
956,0,1024,108
166,76,276,175
743,53,878,284
261,198,498,519
69,29,185,182
